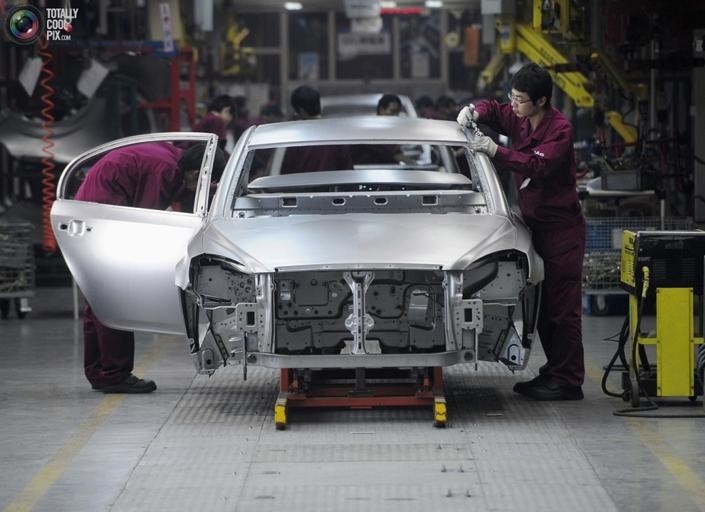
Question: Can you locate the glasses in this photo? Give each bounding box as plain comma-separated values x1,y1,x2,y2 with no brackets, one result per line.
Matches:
507,91,534,105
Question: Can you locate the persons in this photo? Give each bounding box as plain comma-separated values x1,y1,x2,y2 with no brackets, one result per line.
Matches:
60,140,229,393
190,92,238,157
259,87,284,119
453,62,589,400
434,94,463,117
228,96,261,135
360,93,420,167
290,85,356,169
417,98,439,120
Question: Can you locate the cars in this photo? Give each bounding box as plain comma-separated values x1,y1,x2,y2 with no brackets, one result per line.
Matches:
50,116,545,381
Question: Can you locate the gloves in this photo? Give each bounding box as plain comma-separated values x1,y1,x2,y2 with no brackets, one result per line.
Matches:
469,135,499,159
456,105,479,128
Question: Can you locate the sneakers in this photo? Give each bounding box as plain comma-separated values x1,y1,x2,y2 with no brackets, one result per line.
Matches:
91,375,157,395
511,373,585,401
